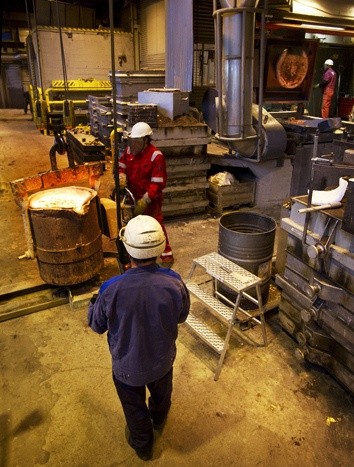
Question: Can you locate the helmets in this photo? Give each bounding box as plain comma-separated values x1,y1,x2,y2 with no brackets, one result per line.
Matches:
324,59,334,66
129,122,153,138
122,214,166,259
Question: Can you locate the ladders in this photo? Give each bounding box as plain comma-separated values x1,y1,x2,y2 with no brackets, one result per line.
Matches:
186,250,269,382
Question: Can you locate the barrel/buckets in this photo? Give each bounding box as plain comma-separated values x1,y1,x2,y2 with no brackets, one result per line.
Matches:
213,211,277,310
28,185,104,287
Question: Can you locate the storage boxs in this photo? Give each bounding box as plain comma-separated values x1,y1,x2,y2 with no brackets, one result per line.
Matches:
208,178,255,217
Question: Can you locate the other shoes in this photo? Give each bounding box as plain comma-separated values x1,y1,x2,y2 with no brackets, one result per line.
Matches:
125,427,153,461
159,261,173,269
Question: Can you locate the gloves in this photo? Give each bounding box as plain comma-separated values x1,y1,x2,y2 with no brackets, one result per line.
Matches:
119,173,127,190
133,198,147,216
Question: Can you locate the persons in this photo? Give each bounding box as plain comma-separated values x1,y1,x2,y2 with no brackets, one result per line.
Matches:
23,93,30,115
88,215,191,461
119,122,175,269
314,59,337,118
